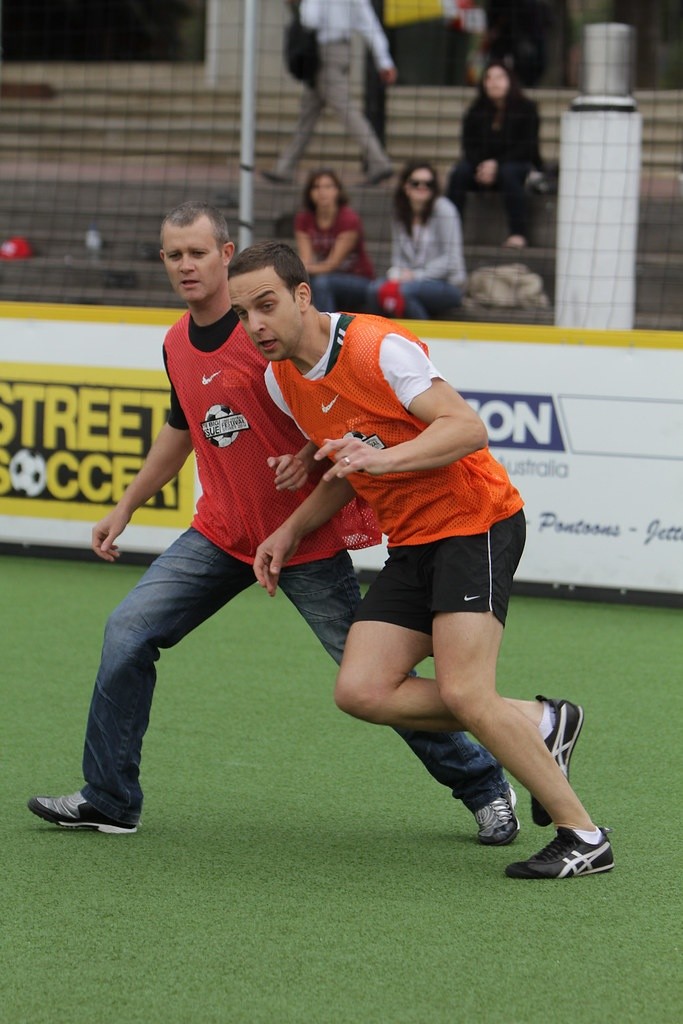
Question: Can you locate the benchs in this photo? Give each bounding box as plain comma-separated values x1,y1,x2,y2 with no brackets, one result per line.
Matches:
1,178,682,329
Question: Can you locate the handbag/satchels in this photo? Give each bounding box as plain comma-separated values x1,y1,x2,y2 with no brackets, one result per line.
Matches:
467,262,549,310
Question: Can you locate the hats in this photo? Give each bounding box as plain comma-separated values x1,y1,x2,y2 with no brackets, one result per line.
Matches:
378,280,405,317
0,237,33,260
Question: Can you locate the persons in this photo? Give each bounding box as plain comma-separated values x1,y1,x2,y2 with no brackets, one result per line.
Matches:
261,0,397,187
226,242,614,879
443,62,555,249
26,200,520,844
293,170,374,312
362,161,468,319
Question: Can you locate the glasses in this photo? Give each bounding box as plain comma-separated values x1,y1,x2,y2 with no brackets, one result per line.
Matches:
408,176,434,188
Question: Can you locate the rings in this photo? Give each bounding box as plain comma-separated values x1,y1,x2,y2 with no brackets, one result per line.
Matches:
344,457,350,465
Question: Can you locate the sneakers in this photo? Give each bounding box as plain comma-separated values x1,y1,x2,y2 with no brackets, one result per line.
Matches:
504,825,614,880
27,790,139,834
529,695,585,826
475,782,521,846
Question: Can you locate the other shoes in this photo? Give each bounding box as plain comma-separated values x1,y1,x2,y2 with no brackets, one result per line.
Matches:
360,169,393,187
506,237,526,248
262,171,296,185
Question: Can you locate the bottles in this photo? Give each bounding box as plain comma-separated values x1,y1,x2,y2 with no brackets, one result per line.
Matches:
84,224,101,271
